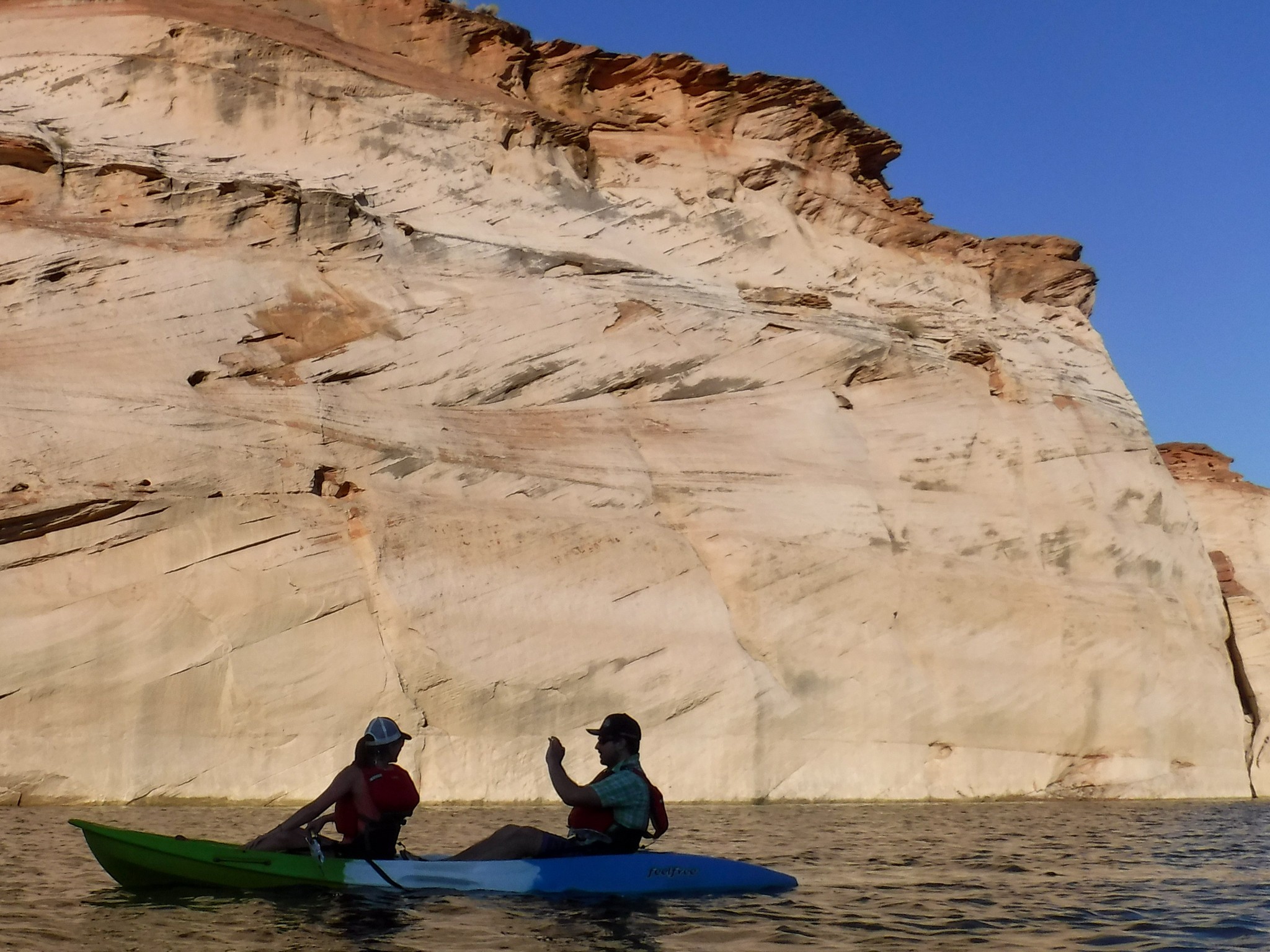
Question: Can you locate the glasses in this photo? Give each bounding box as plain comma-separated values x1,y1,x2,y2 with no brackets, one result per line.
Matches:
598,738,609,745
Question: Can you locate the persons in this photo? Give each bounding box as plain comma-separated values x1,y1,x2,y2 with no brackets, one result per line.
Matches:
400,713,652,862
175,715,413,860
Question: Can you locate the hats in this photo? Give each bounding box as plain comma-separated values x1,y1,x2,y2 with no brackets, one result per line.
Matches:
365,716,412,746
585,713,641,741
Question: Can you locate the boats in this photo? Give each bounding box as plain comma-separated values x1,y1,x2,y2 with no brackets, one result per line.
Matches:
65,816,799,900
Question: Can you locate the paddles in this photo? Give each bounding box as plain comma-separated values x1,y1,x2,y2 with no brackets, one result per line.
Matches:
305,825,325,864
